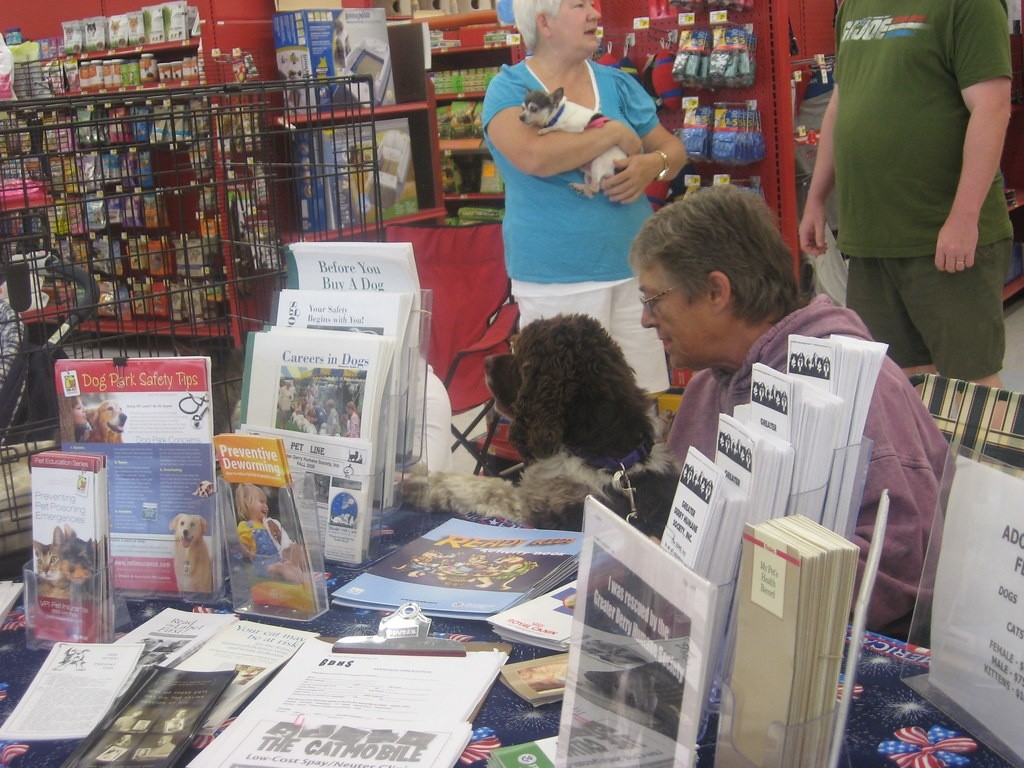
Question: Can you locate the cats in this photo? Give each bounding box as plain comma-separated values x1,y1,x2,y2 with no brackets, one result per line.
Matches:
334,20,351,63
32,527,73,599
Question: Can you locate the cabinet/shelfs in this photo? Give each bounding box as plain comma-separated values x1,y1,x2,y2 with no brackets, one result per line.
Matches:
0,0,788,348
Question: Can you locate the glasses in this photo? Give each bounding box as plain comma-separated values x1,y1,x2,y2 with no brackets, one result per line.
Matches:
639,284,680,317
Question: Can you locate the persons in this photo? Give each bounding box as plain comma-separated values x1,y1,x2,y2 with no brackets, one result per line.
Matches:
114,710,144,731
480,1,689,396
278,376,360,438
628,184,955,648
236,483,305,584
164,708,189,733
71,396,90,442
143,735,176,759
104,735,132,750
798,0,1014,390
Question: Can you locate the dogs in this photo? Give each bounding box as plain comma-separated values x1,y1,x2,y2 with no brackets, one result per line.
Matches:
59,524,100,597
396,313,681,541
169,512,212,593
290,403,318,435
518,86,629,199
85,399,127,443
128,17,139,33
86,22,96,37
191,480,215,498
64,26,74,41
110,19,121,34
262,517,309,574
346,145,370,216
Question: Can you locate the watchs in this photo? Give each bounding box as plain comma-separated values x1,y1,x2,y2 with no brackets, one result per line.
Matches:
654,150,669,181
